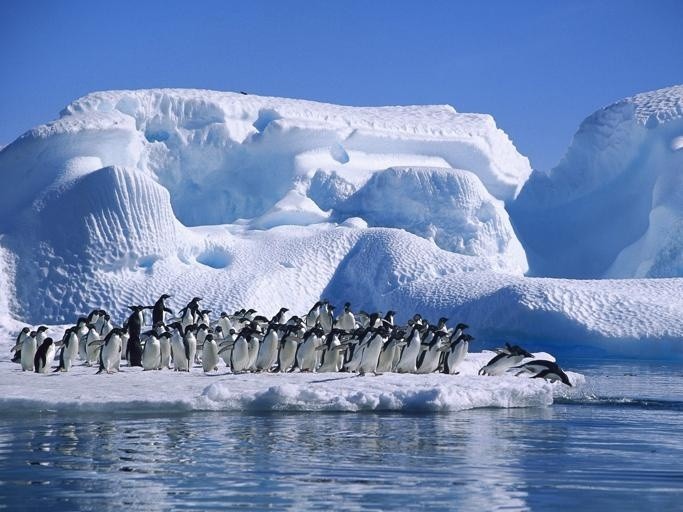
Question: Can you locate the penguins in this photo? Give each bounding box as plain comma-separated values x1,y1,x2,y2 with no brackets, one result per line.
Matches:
9,294,476,375
478,341,573,387
